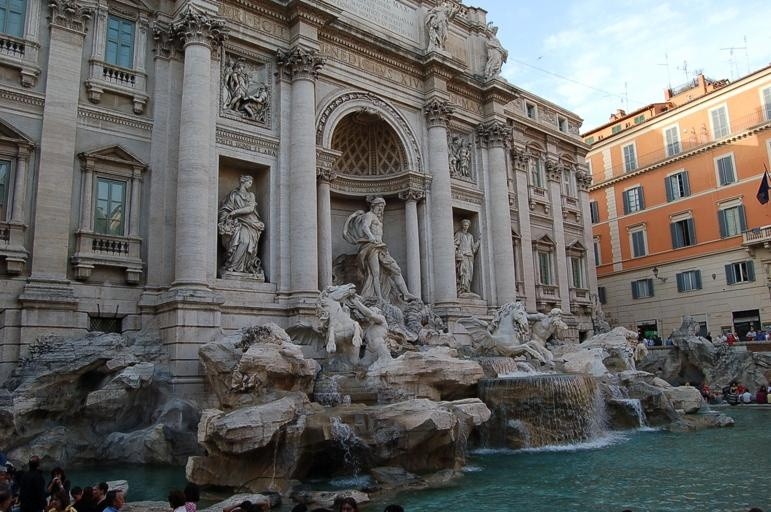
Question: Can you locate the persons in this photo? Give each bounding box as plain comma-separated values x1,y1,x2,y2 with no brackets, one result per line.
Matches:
695,379,771,406
484,22,510,79
0,454,124,512
447,135,472,177
243,82,269,121
640,334,671,345
225,57,237,108
423,0,462,54
454,218,482,295
703,324,771,345
219,174,264,282
228,62,247,114
168,484,405,512
527,308,570,366
351,296,395,369
361,196,420,304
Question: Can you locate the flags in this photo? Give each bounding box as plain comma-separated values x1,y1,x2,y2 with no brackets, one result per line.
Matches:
755,170,771,205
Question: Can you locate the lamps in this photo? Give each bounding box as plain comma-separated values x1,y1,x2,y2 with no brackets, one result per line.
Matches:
652,267,667,282
